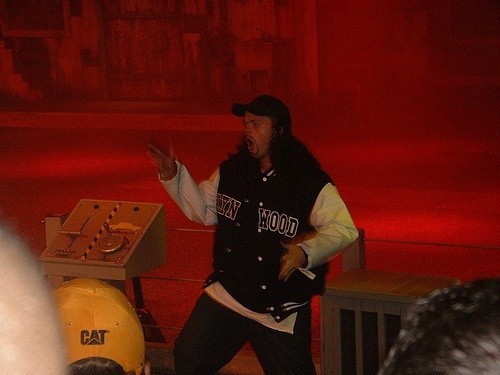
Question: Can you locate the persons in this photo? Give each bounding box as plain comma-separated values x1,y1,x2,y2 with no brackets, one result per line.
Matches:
141,95,360,375
379,275,500,375
49,277,151,375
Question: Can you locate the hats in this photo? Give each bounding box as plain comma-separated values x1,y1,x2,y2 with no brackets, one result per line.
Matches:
232,95,290,124
